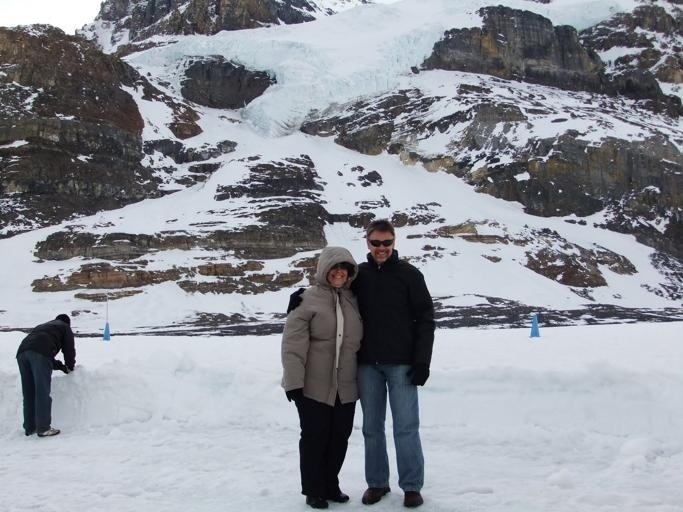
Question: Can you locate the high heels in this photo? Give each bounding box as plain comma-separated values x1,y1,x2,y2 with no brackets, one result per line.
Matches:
527,313,540,339
101,322,113,341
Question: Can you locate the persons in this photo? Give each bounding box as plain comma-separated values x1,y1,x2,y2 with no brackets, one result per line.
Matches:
16,314,77,437
285,218,437,509
275,246,364,510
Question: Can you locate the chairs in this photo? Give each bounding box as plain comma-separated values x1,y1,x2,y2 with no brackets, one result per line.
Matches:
38,428,60,437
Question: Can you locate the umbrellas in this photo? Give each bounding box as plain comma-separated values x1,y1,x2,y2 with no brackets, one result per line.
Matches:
306,488,423,508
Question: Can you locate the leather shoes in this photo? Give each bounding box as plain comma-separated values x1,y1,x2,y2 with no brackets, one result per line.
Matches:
288,292,303,313
286,389,310,411
408,364,429,386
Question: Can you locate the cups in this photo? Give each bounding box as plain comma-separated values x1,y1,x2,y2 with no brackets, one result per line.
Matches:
331,263,349,270
367,236,394,247
